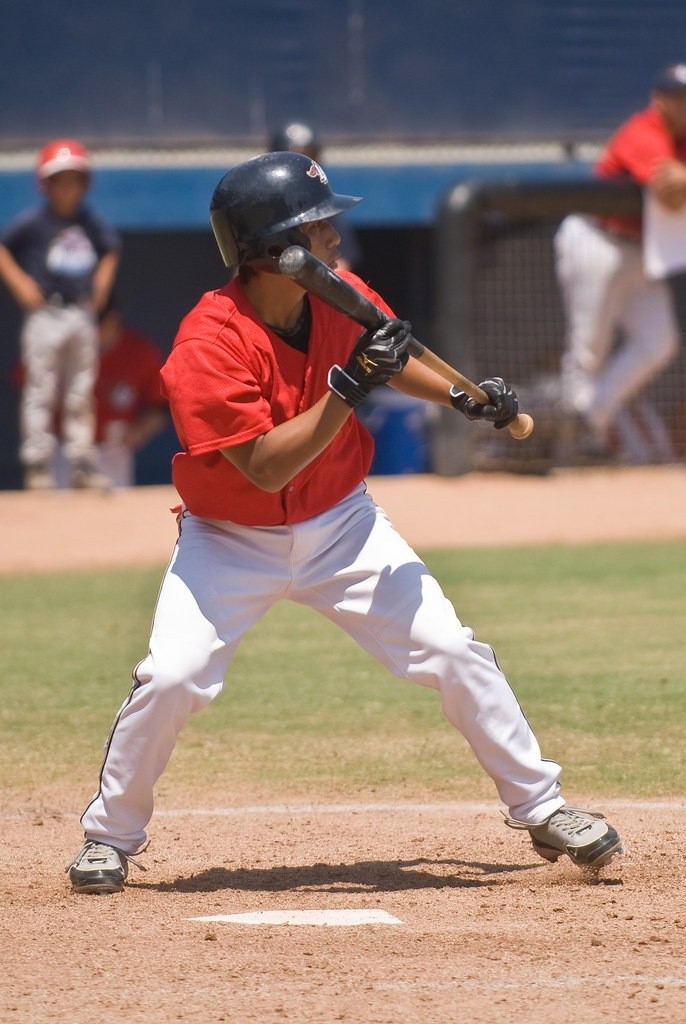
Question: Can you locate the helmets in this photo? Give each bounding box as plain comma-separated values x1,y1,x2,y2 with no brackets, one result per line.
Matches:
211,151,362,272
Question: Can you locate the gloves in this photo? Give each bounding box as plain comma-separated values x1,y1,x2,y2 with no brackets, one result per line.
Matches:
327,320,415,408
450,377,518,429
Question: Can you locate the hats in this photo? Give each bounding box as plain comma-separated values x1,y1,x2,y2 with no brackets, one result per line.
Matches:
656,64,686,93
37,140,91,183
270,122,319,159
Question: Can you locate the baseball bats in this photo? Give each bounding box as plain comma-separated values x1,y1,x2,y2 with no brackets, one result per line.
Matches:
279,241,539,443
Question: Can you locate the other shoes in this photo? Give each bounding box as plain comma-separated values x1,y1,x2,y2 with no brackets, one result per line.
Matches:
24,464,59,489
565,414,622,466
70,462,112,490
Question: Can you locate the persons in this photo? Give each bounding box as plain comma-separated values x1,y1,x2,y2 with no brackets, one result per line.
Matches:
553,62,686,463
66,152,621,895
0,139,173,491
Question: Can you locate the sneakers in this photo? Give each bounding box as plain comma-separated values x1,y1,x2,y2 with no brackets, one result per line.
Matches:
501,805,622,868
65,839,152,893
